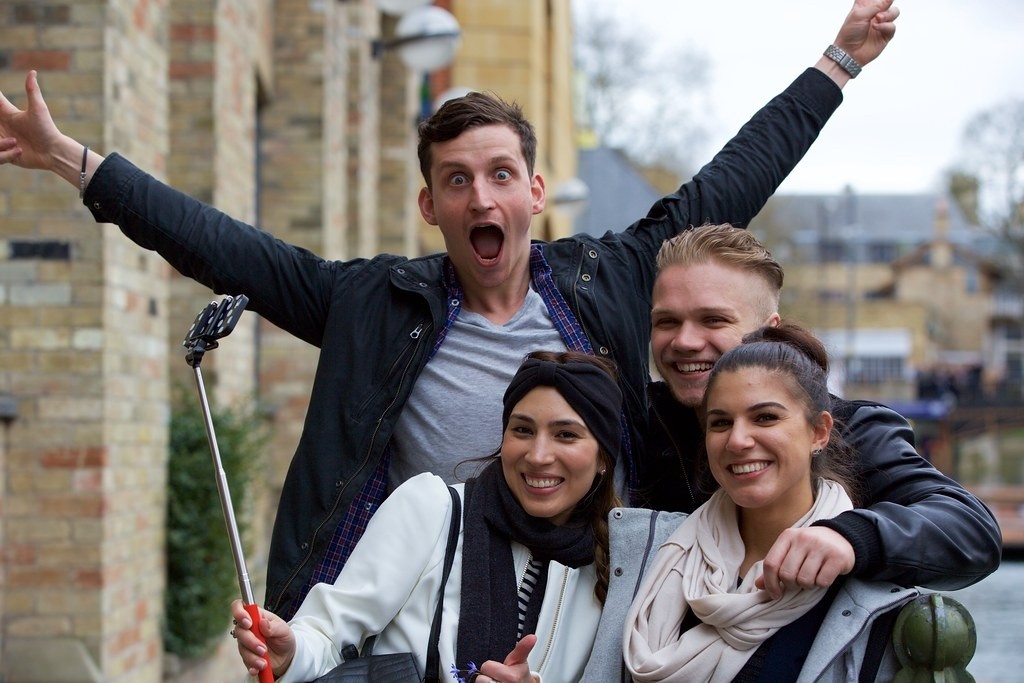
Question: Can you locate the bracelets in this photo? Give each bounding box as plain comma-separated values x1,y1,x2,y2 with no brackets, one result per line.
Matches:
79,146,87,199
823,45,862,79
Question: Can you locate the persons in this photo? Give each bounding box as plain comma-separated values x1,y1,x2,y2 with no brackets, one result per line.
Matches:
580,321,918,683
0,0,901,622
629,223,1002,599
230,351,625,682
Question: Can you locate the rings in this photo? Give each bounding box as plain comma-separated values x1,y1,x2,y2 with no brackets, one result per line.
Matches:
229,619,238,639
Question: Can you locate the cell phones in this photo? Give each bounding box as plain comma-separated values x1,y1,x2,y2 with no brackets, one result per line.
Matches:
184,295,249,345
